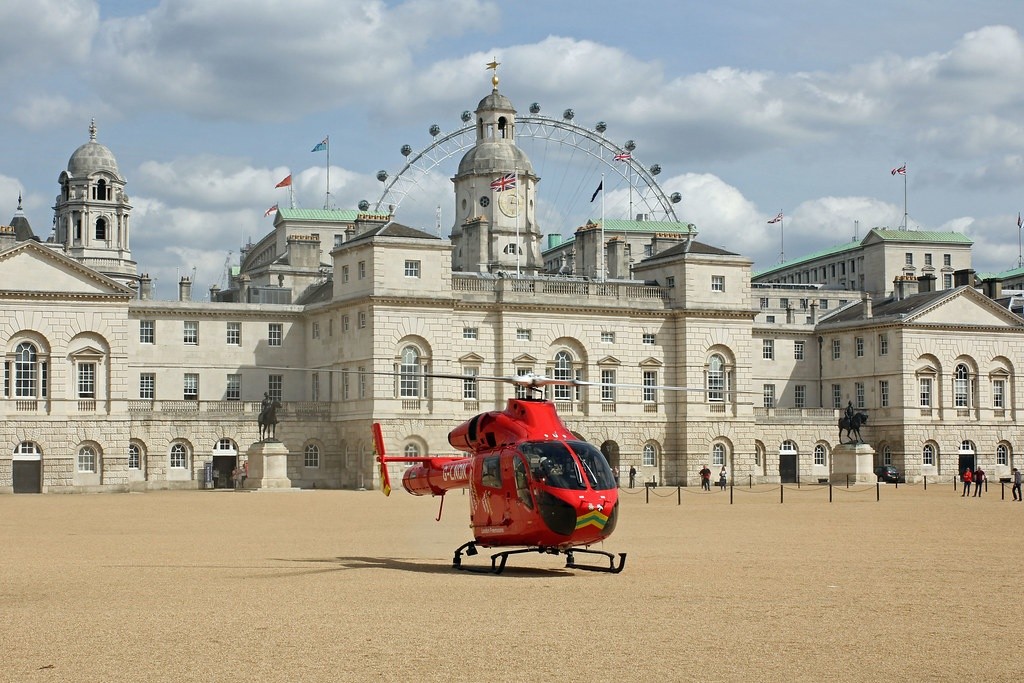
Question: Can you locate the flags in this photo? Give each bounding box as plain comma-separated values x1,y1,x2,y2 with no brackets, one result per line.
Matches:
591,181,602,202
613,154,629,162
892,164,907,176
768,214,783,224
490,172,516,192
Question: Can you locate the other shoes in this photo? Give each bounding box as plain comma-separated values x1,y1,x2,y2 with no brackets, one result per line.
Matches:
1017,499,1022,501
1012,498,1017,501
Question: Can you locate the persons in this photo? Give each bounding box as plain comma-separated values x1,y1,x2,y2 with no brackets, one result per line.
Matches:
961,468,972,497
261,391,279,423
845,401,854,429
212,467,243,488
972,466,986,497
719,466,727,491
628,465,637,489
612,466,619,488
1012,468,1022,501
699,464,711,491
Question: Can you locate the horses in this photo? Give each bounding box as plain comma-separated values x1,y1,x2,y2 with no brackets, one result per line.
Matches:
839,412,869,444
258,400,282,442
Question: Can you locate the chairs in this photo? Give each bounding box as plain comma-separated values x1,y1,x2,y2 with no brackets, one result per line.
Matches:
483,474,501,488
515,470,533,509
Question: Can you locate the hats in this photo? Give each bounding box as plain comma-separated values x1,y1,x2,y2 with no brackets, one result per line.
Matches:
966,468,970,471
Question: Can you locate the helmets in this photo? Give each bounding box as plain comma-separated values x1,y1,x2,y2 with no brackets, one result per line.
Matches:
540,458,553,473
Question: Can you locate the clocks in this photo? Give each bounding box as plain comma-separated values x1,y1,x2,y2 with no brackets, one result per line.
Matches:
499,189,524,217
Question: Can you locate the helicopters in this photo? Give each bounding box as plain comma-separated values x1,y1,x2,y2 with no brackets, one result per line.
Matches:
241,365,763,575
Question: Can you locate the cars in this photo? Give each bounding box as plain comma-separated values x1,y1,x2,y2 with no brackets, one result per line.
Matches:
874,466,900,483
